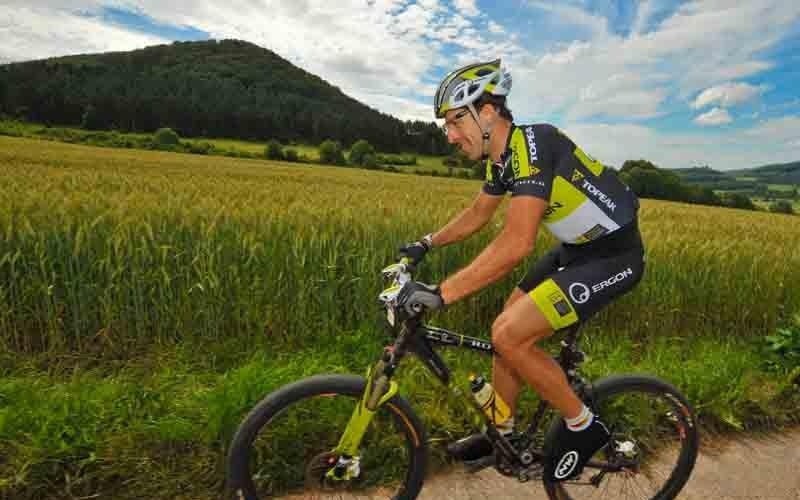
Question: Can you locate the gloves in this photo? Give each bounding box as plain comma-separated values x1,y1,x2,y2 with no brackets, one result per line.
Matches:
396,278,444,316
394,233,434,268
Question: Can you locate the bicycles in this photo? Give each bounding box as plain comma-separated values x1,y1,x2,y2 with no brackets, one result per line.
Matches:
226,248,698,500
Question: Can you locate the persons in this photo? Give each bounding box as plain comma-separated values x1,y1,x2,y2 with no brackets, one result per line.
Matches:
391,59,645,481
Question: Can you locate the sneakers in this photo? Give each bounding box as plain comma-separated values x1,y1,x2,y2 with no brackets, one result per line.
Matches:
545,417,613,483
449,425,516,466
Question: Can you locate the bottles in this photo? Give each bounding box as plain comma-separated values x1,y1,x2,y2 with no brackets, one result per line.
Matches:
468,374,512,425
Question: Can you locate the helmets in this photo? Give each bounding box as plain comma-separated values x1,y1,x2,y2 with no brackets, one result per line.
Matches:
434,57,514,116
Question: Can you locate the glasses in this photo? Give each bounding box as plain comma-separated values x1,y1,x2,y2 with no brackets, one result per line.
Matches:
437,109,472,134
